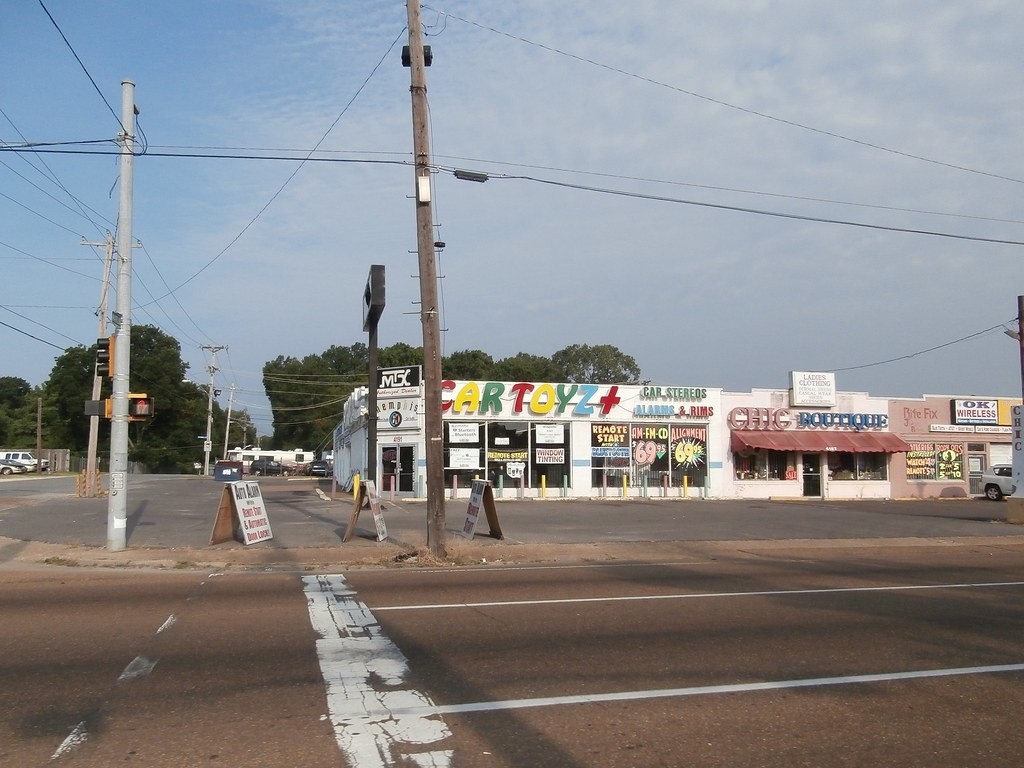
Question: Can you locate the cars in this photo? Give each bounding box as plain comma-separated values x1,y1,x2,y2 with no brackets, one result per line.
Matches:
305,460,329,476
0,459,35,475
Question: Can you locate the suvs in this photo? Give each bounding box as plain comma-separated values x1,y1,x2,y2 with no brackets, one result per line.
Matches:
250,460,296,477
977,463,1015,501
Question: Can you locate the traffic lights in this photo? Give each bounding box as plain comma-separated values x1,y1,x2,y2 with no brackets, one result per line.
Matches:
96,335,116,379
129,397,155,418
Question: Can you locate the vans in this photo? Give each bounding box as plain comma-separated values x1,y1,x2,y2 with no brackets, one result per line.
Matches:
0,451,50,471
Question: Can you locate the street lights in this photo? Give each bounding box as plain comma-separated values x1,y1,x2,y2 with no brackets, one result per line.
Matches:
228,420,246,450
182,380,213,476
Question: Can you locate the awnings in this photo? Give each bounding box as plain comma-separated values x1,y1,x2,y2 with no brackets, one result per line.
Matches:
731,430,912,455
